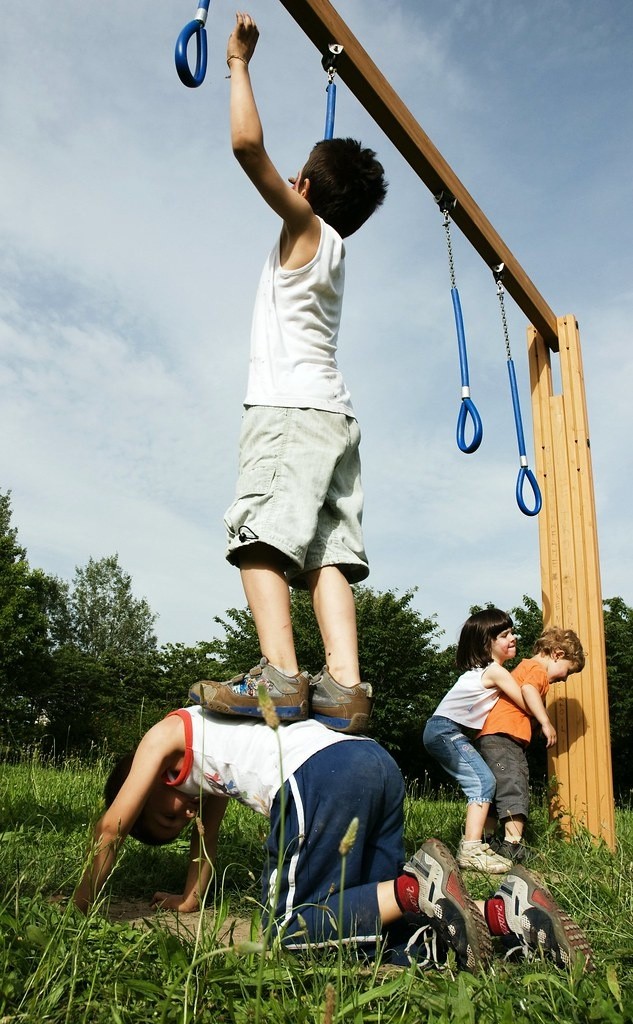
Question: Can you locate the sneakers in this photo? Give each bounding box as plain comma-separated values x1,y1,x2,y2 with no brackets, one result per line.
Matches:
309,665,374,733
188,657,312,721
404,837,493,979
495,865,597,975
455,843,513,874
484,835,535,860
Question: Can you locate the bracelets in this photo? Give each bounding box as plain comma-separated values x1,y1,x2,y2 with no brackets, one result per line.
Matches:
225,55,247,79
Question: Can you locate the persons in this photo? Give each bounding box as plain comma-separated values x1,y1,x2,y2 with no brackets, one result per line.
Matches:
473,626,585,860
423,610,552,873
187,11,388,732
56,704,591,978
